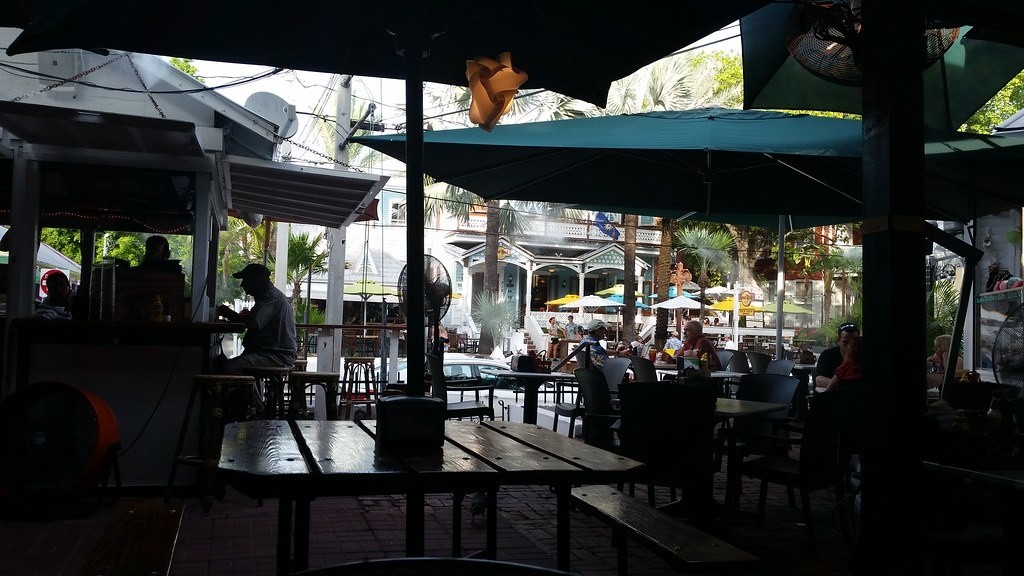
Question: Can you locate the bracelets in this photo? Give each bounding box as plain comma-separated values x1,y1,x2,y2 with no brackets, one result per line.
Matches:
617,351,619,356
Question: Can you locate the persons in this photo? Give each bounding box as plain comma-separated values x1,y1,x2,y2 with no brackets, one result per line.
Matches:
710,317,722,347
794,345,816,396
662,331,682,351
131,236,185,322
702,318,711,346
564,315,578,340
661,321,723,374
39,273,76,308
432,322,450,353
826,335,863,390
814,323,861,387
217,263,298,416
547,316,566,361
927,334,952,373
578,319,637,408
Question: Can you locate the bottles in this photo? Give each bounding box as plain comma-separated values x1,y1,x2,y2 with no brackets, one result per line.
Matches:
150,296,163,322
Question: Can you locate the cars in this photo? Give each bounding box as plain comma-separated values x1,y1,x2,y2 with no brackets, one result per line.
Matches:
348,352,579,417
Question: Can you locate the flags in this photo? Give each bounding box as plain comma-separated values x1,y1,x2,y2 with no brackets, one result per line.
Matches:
592,212,620,239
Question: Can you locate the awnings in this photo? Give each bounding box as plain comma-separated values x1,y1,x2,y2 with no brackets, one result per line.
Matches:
228,154,390,231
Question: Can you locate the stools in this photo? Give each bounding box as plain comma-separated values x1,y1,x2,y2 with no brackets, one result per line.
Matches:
161,355,382,517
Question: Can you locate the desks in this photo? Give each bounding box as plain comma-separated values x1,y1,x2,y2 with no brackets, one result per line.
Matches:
656,368,748,390
217,416,644,576
718,398,787,504
482,369,575,424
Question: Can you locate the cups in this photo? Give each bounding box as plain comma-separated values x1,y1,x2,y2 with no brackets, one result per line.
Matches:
164,315,171,323
55,306,65,314
649,349,656,362
209,307,216,322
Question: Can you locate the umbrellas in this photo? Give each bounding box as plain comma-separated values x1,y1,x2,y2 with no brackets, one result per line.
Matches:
262,198,381,230
343,108,1024,361
12,0,772,558
544,283,816,342
343,278,403,337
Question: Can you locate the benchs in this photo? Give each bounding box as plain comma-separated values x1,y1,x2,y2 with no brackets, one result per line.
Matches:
567,483,762,576
79,499,186,576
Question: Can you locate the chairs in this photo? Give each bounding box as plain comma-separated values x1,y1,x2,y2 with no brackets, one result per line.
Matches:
424,351,871,544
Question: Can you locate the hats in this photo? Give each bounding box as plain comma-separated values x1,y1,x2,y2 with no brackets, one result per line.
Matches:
588,319,611,332
232,264,271,279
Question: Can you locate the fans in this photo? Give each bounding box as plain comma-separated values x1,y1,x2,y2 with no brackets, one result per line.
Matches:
991,305,1024,415
783,0,978,89
398,253,452,350
